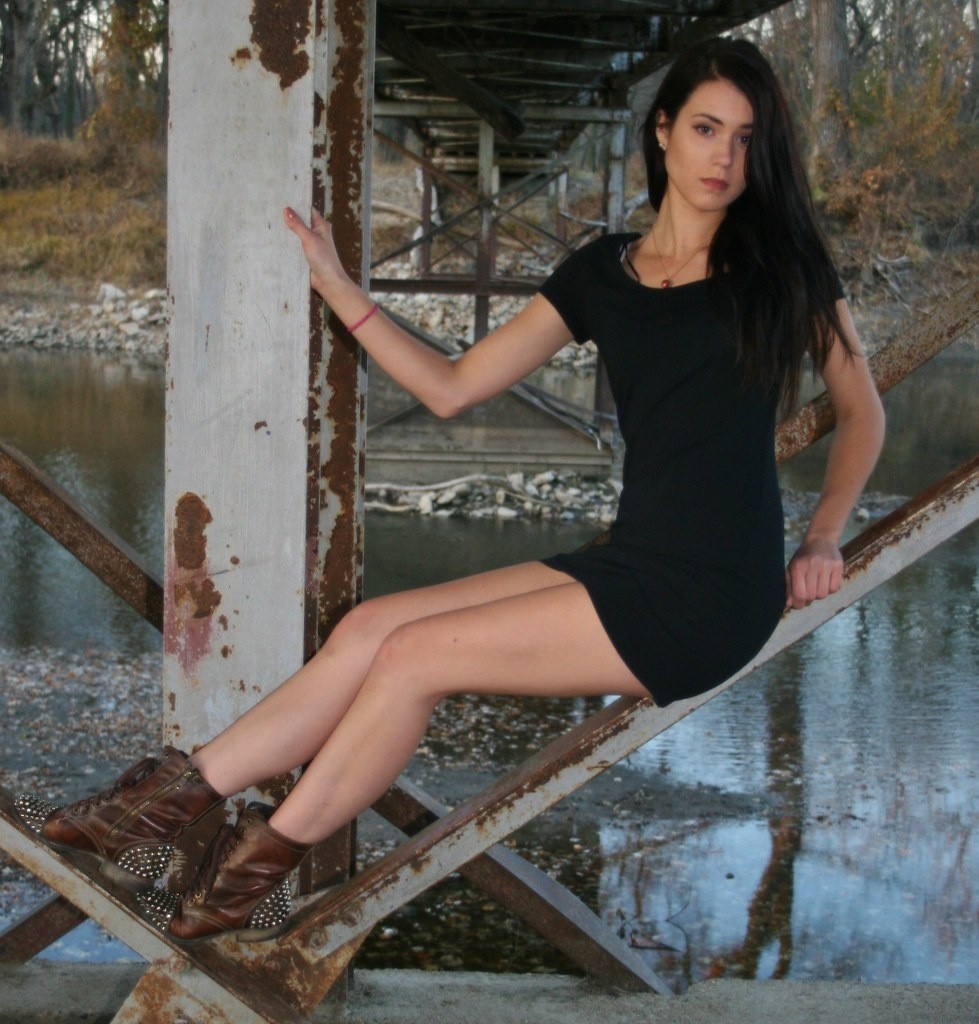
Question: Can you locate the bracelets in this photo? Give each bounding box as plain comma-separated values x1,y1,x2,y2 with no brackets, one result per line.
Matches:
344,302,380,335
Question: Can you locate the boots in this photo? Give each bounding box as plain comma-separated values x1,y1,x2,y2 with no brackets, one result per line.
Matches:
13,743,225,894
136,819,315,944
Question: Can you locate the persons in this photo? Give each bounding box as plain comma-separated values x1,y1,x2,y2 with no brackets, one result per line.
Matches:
13,34,888,942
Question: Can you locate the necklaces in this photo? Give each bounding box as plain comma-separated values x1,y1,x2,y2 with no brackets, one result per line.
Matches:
651,223,711,290
